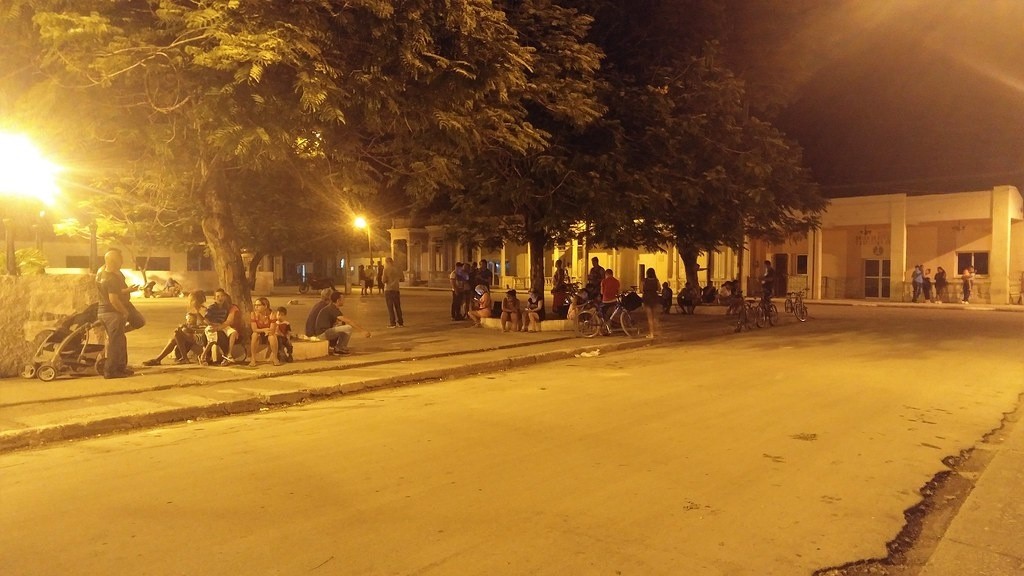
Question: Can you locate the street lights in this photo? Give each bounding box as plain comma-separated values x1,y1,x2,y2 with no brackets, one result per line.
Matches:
353,216,373,260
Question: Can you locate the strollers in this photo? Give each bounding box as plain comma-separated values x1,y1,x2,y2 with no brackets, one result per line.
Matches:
18,301,108,382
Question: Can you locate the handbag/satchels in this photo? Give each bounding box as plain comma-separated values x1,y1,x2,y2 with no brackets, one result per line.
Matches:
621,291,643,311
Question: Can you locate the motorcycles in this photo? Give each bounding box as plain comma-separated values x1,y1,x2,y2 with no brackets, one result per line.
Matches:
297,273,335,294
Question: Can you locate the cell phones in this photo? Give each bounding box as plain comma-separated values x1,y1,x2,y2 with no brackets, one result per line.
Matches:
133,284,140,288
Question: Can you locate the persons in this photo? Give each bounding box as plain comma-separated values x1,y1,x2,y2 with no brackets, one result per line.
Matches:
358,259,384,295
95,248,145,379
662,282,672,314
643,268,661,338
911,265,977,304
450,259,492,324
521,288,544,333
306,288,371,356
677,280,743,315
144,278,181,297
551,257,620,337
501,289,522,332
382,257,405,328
143,288,293,366
759,261,775,318
467,285,491,328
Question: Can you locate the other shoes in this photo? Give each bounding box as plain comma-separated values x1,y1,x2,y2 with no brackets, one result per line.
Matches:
471,321,481,328
197,352,293,366
503,328,536,332
143,358,161,366
104,370,131,378
328,344,351,355
646,333,654,339
451,314,469,321
387,324,404,328
176,357,190,364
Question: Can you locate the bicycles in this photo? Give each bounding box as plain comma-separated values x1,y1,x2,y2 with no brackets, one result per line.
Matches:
574,294,642,339
458,295,475,317
783,288,811,322
623,285,641,295
742,293,779,330
